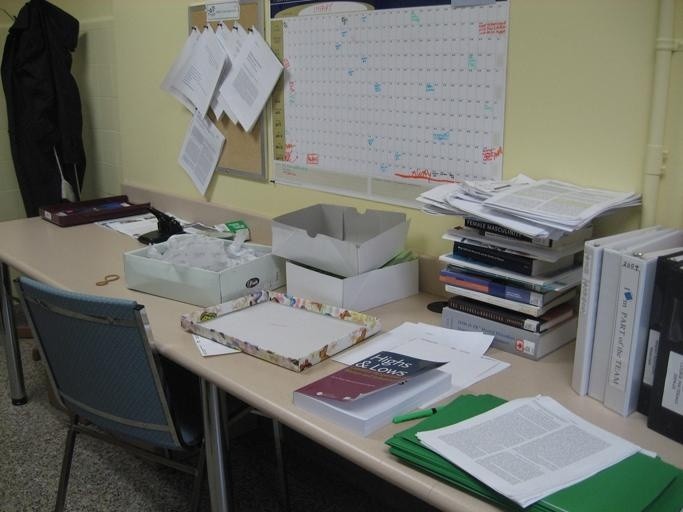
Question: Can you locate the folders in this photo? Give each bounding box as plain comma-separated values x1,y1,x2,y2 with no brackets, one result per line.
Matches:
571,225,683,448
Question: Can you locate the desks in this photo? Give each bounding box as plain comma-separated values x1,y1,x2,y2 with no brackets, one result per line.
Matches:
0,185,683,511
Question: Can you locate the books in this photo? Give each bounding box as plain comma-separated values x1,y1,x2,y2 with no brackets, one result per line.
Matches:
293,351,451,436
435,216,602,361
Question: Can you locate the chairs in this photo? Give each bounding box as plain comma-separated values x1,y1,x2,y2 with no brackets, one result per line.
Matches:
13,276,290,511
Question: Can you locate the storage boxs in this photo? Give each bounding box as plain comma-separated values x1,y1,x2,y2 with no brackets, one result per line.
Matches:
123,235,287,309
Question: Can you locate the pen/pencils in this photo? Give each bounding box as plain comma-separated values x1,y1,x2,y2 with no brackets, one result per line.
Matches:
393,404,446,423
